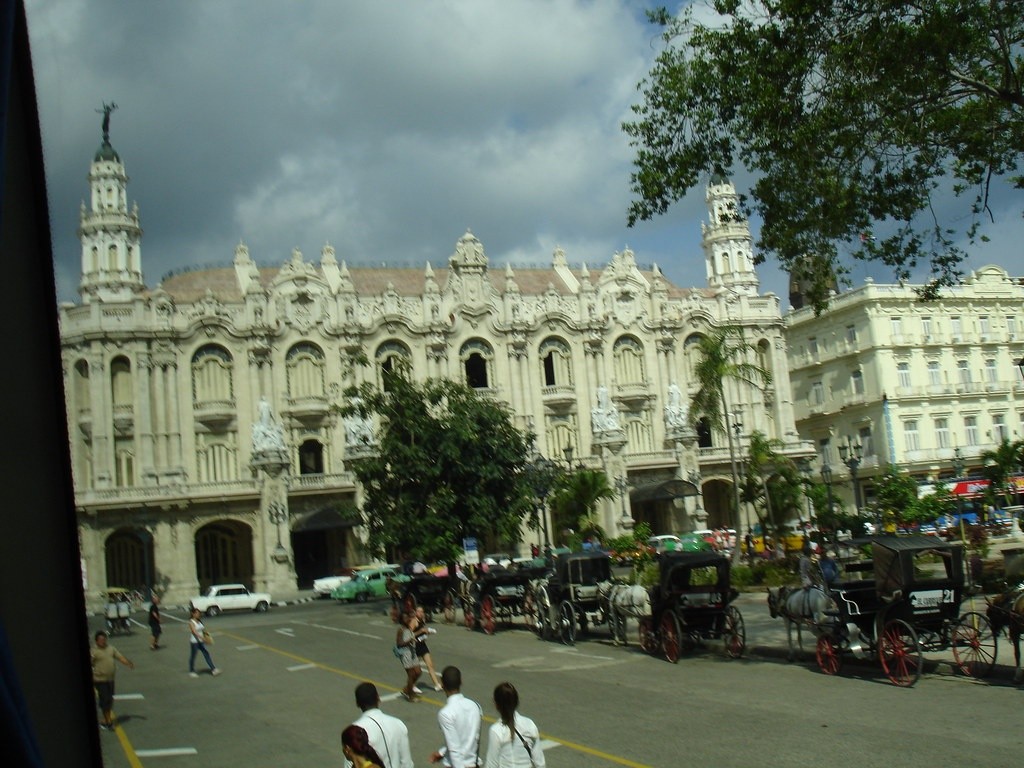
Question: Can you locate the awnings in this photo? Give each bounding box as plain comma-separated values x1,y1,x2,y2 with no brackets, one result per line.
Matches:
628,477,699,506
290,497,364,533
551,490,591,516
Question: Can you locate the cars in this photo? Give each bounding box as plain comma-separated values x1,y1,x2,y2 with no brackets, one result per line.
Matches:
314,562,411,602
752,529,806,554
189,582,272,616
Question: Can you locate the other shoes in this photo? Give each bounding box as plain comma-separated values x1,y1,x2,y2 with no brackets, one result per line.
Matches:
108,723,114,732
100,719,107,728
211,668,223,676
412,685,423,694
189,671,199,678
434,684,443,691
401,690,413,701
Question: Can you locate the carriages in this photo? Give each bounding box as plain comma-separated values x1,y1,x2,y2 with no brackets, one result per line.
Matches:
595,550,746,661
383,571,457,626
767,531,999,688
444,550,615,645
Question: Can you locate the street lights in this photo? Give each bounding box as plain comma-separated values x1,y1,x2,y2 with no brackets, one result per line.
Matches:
837,435,865,517
819,460,833,518
531,456,555,562
562,446,573,474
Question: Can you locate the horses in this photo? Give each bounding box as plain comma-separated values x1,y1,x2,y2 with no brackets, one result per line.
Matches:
382,573,411,612
528,577,549,638
596,578,653,646
765,586,840,664
985,583,1024,683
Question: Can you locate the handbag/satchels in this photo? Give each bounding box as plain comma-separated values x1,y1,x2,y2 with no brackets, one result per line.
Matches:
203,631,214,645
393,645,405,657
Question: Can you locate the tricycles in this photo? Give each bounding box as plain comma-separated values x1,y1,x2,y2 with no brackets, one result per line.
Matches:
97,587,135,636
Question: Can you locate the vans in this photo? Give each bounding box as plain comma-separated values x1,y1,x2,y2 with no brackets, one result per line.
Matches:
646,535,681,552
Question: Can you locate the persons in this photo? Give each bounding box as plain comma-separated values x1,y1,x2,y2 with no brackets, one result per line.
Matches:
597,382,607,411
665,379,681,408
341,681,415,768
105,594,130,628
485,682,546,768
430,665,484,768
89,631,134,732
148,594,162,650
804,508,896,554
970,550,983,584
257,395,275,427
800,547,841,586
713,525,777,559
397,606,442,699
530,543,551,559
188,609,221,678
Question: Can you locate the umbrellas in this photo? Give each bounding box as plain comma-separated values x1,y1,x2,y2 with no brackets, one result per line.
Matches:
101,587,129,593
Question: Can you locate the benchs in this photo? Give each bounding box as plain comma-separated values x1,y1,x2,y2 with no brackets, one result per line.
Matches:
838,582,878,618
